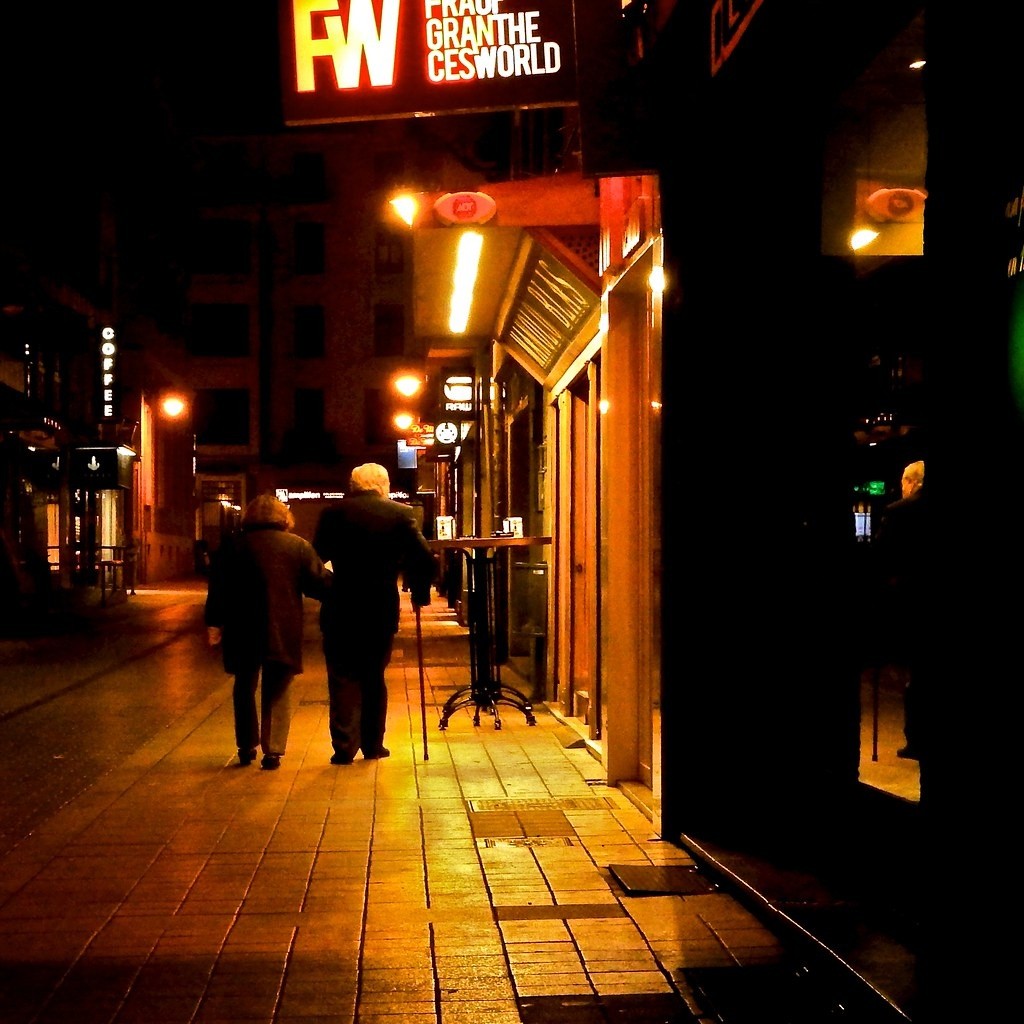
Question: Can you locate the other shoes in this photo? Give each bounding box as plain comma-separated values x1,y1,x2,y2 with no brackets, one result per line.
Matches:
239,749,257,767
331,753,353,765
261,755,279,769
364,747,390,759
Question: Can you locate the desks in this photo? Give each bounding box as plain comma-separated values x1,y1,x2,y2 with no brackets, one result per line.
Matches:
425,532,552,730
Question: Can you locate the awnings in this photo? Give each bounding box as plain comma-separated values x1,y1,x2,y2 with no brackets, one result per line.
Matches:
0,381,60,431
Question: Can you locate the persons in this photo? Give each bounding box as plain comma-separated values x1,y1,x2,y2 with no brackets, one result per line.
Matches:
309,463,438,764
204,495,331,767
872,459,925,760
